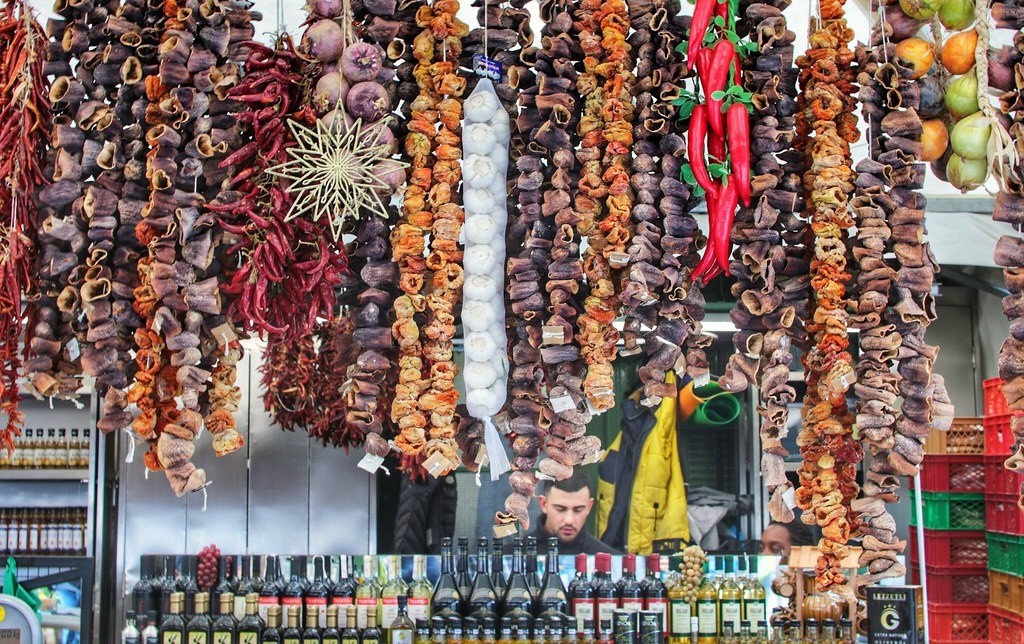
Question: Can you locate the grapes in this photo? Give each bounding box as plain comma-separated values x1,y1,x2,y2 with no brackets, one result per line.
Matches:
679,545,707,605
198,545,218,591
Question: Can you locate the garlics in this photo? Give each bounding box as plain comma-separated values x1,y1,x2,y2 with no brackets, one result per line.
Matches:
463,87,509,420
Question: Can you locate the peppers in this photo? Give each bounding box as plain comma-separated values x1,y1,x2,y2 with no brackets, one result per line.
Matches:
204,26,394,462
1,0,52,452
672,0,753,282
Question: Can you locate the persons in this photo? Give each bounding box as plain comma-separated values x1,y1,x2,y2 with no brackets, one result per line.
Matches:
499,472,625,557
758,519,815,562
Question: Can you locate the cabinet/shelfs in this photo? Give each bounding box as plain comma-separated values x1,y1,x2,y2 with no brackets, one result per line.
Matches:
0,381,120,644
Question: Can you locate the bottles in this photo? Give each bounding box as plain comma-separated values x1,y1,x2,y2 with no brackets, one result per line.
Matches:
122,534,858,644
0,420,92,471
1,502,87,554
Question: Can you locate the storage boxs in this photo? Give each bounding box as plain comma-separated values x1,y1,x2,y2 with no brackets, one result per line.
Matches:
985,532,1024,579
928,601,989,644
982,415,1015,455
984,494,1024,536
921,416,985,455
909,559,989,603
908,488,986,531
986,604,1024,644
983,455,1024,496
908,525,988,569
982,377,1017,416
987,569,1024,619
908,455,986,493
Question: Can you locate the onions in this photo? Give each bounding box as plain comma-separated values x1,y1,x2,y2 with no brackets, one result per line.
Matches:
302,0,406,201
